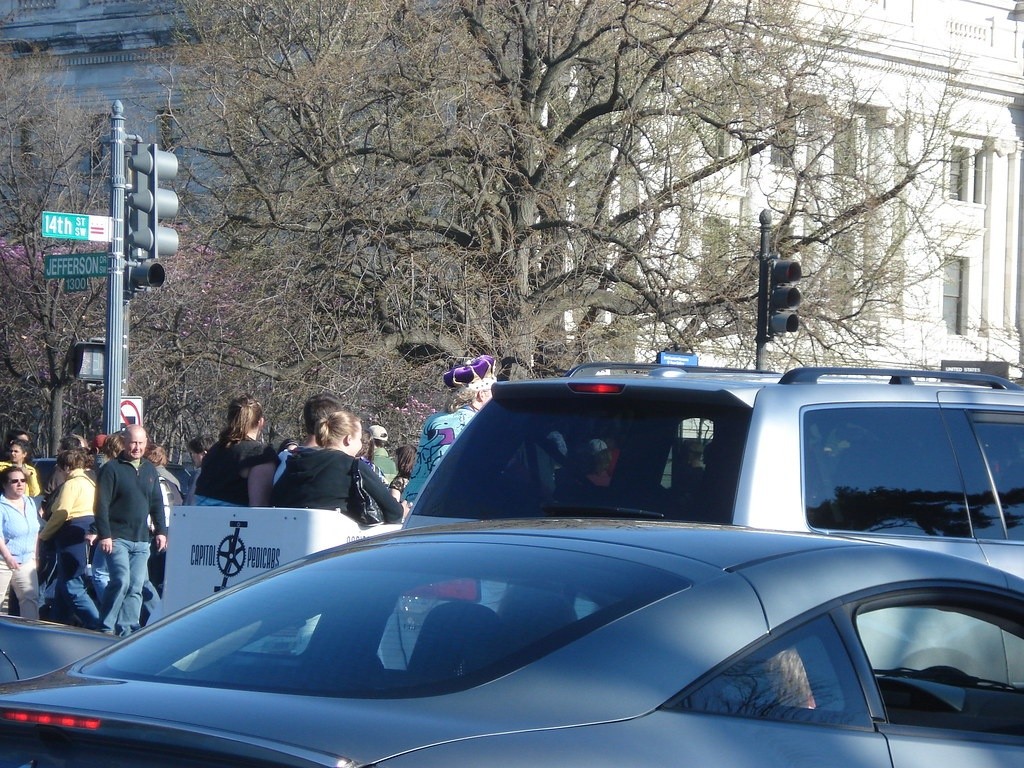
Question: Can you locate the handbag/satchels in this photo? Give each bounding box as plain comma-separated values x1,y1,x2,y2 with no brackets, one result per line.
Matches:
347,458,385,527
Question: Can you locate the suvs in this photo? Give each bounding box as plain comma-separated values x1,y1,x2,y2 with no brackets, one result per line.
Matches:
399,363,1024,585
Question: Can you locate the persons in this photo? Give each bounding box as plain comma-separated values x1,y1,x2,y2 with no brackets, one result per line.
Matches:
273,411,409,524
278,439,299,454
356,425,417,503
763,638,842,712
274,393,342,482
194,395,282,507
401,355,554,505
556,427,712,500
184,435,216,506
0,424,185,637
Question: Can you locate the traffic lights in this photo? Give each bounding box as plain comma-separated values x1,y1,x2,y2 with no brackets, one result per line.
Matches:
768,260,803,334
73,341,107,381
128,142,179,261
125,262,166,292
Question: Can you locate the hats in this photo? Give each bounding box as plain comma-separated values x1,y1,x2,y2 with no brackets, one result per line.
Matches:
443,355,497,394
91,435,107,449
370,425,388,441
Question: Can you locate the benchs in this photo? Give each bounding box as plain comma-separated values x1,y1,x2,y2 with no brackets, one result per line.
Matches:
162,505,404,620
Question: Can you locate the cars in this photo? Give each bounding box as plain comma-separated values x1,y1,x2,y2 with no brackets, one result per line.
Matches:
0,518,1024,768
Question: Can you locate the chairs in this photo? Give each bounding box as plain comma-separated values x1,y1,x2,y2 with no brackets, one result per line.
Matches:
407,595,510,695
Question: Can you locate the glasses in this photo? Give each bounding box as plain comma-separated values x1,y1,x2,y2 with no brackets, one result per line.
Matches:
11,479,26,483
